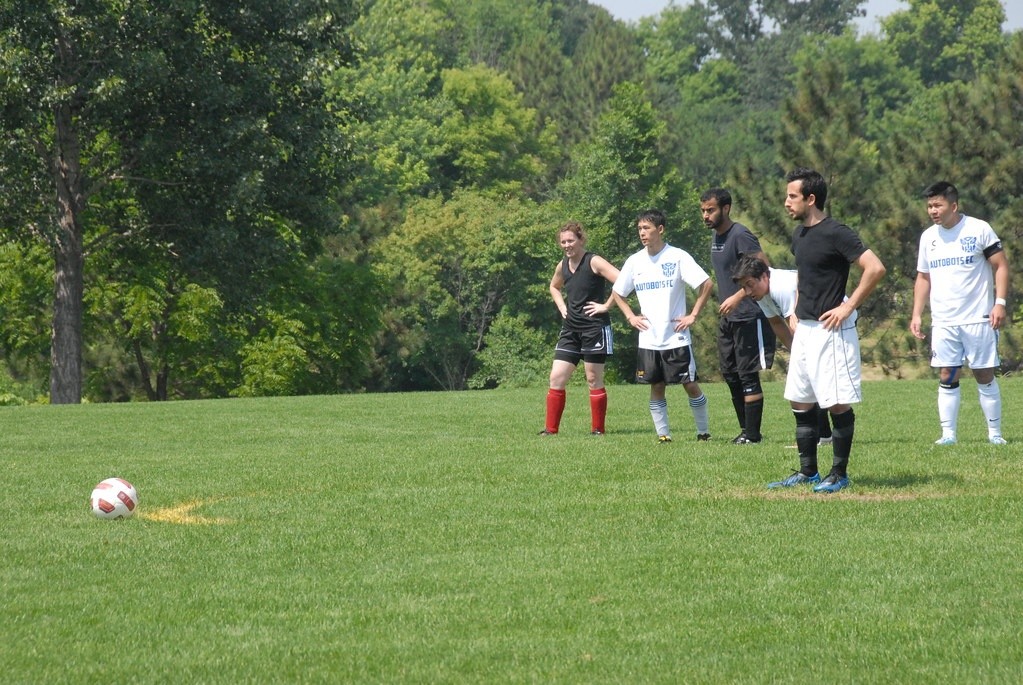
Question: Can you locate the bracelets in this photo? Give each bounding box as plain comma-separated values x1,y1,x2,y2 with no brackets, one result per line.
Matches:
995,297,1007,307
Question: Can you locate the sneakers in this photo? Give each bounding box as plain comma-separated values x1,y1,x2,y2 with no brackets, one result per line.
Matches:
732,429,746,445
657,435,671,445
745,433,763,445
934,437,956,445
813,471,847,493
697,432,711,443
767,469,821,490
988,435,1008,445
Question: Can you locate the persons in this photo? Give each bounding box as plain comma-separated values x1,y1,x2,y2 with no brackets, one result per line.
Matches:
731,167,886,494
613,210,714,445
910,182,1009,445
700,188,776,444
536,222,620,436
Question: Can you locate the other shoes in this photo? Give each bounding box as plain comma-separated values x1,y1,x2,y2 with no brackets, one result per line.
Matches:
537,430,552,436
592,428,603,435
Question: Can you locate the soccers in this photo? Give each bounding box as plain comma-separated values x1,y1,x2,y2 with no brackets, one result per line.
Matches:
89,478,140,520
